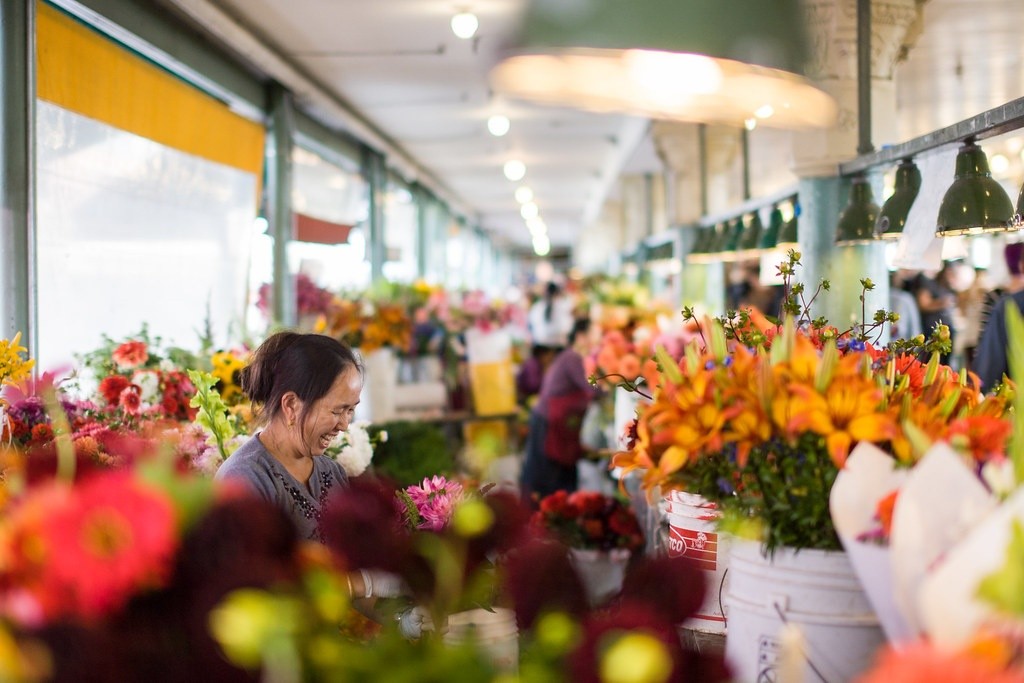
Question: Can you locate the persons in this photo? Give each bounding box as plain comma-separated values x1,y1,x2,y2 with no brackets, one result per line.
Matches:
212,330,433,643
513,282,610,496
725,243,1024,409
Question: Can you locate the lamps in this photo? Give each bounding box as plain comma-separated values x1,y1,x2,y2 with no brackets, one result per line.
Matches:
485,0,835,131
872,157,923,242
622,194,798,269
835,175,881,248
935,141,1016,237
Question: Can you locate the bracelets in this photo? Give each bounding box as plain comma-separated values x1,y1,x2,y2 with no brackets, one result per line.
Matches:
346,575,354,601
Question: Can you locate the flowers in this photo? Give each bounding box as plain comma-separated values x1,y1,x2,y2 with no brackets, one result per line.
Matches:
0,250,1024,683
